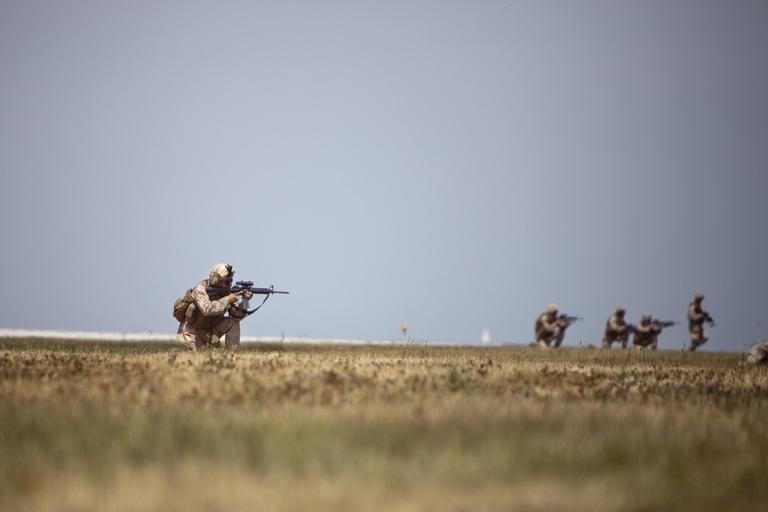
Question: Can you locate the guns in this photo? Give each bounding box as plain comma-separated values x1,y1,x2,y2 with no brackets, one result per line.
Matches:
206,280,289,297
697,308,719,328
552,313,585,326
652,318,681,328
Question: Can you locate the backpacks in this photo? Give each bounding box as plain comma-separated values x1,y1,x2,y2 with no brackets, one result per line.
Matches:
173,288,197,322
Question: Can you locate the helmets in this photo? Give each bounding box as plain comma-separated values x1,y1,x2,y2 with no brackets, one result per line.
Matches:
547,303,558,313
642,313,651,323
615,306,625,313
694,292,704,299
209,262,234,286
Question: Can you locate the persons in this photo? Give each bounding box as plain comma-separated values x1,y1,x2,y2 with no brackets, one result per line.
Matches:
529,303,571,348
634,313,662,351
173,263,252,352
588,305,630,349
687,291,709,351
